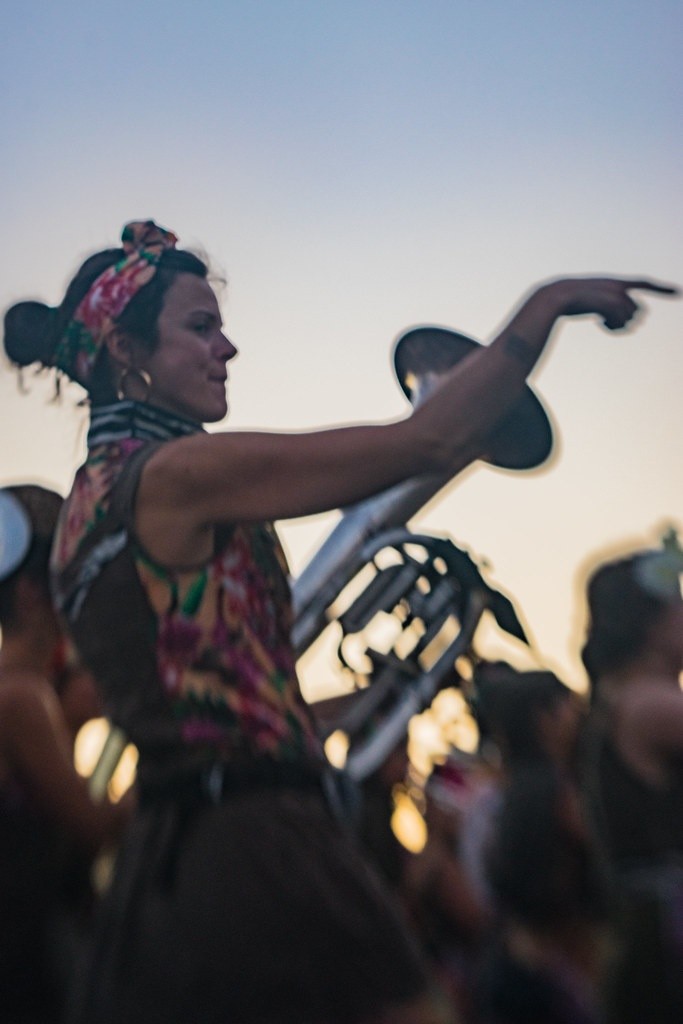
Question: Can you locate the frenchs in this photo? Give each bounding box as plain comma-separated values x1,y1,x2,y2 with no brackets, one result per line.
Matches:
85,323,555,827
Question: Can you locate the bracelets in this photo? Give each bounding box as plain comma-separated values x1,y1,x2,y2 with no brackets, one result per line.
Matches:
500,319,543,374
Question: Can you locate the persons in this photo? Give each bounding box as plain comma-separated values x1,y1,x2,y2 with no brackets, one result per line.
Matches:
0,480,138,1023
1,207,682,1024
399,527,682,1024
